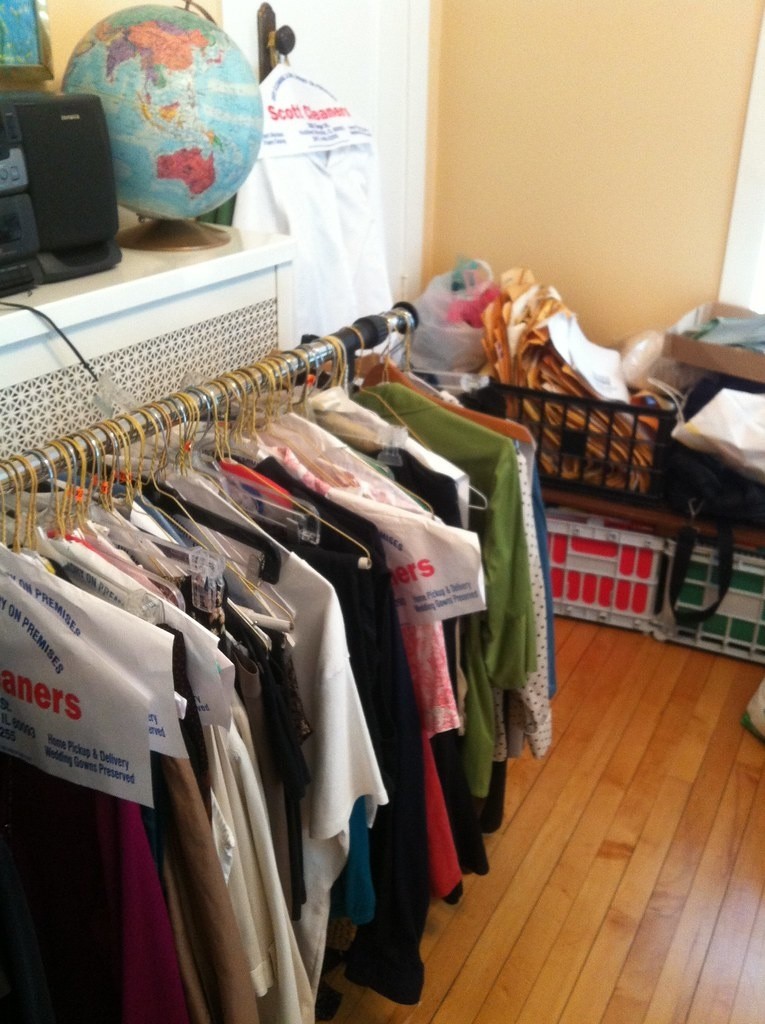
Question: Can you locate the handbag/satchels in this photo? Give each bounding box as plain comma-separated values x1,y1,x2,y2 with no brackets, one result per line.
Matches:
646,386,765,486
650,370,765,624
405,255,495,372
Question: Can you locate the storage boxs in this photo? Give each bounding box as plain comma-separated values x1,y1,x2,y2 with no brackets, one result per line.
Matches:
473,302,765,665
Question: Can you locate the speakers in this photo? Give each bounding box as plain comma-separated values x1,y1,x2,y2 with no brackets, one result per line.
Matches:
14,92,121,283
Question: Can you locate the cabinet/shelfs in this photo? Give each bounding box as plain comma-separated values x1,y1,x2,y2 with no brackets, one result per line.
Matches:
0,224,302,457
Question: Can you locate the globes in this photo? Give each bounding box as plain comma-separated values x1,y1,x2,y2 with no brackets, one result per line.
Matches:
60,1,265,253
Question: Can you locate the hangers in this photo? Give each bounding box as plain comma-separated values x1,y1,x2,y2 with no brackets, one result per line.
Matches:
1,311,531,716
